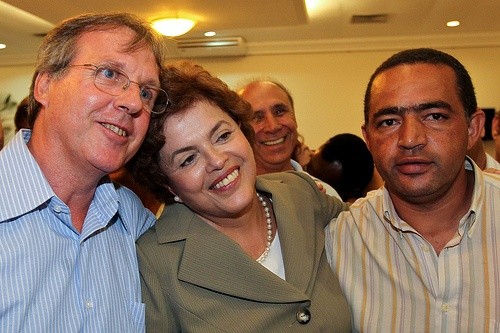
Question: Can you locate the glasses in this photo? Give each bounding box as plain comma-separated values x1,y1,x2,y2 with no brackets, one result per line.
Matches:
68,62,170,115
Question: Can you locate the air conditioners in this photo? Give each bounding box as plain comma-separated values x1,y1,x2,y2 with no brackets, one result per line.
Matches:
176,36,248,57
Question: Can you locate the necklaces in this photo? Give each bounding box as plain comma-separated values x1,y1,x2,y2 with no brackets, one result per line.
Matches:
256,192,272,264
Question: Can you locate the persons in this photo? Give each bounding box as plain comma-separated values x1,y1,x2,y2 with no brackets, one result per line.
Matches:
324,49,500,333
235,79,342,200
134,61,357,333
0,12,326,333
312,134,374,202
